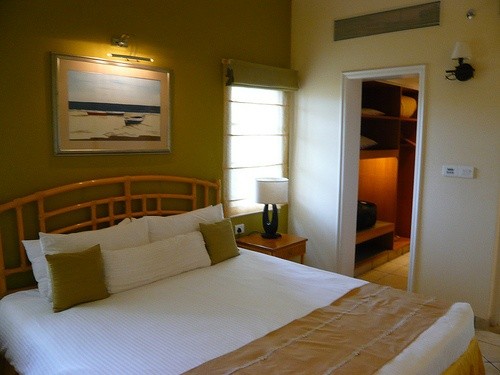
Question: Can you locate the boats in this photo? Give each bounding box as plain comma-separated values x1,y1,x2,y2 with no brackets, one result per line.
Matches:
87,111,124,116
125,114,145,125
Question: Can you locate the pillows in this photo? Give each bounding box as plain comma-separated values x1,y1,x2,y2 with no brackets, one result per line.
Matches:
199,217,240,264
21,218,131,299
144,203,224,243
102,232,211,294
361,109,385,116
39,218,150,303
360,136,378,150
45,244,111,313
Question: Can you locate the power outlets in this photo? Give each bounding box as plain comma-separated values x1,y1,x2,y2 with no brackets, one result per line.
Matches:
235,225,244,234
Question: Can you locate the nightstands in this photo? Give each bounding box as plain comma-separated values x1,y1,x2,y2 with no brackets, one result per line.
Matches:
237,232,308,264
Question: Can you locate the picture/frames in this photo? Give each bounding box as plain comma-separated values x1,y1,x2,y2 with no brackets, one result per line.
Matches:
50,51,175,156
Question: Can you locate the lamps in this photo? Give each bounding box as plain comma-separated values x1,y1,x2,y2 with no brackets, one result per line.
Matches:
108,33,155,62
444,41,475,82
256,177,288,239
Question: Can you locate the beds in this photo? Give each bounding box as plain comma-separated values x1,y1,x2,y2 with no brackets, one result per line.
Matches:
0,176,486,375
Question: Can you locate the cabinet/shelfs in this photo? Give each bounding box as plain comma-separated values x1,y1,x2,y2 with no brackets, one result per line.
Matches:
355,80,418,238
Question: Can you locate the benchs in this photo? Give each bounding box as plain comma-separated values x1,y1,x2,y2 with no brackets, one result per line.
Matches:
355,221,395,250
